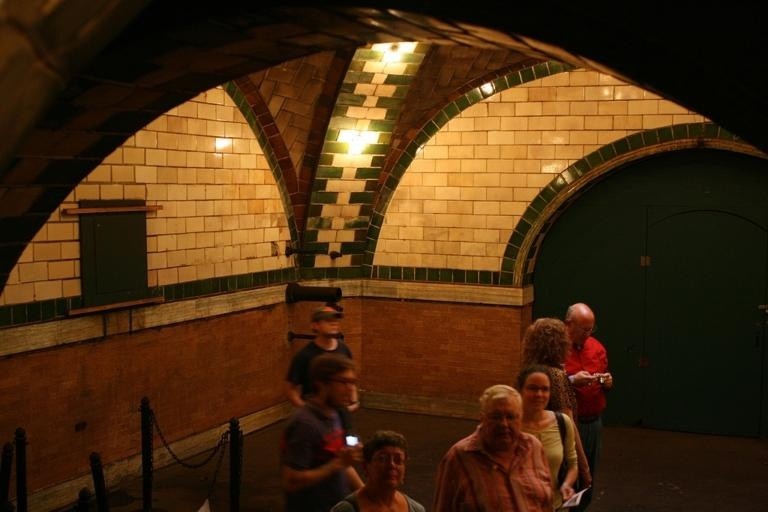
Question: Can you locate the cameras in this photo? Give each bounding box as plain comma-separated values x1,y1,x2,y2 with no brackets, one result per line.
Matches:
592,373,607,385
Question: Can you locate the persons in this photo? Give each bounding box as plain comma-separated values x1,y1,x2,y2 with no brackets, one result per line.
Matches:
330,430,425,512
278,353,365,512
518,303,614,512
433,384,554,512
283,306,361,413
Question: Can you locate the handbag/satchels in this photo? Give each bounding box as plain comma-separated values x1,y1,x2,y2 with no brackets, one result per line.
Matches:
557,412,592,512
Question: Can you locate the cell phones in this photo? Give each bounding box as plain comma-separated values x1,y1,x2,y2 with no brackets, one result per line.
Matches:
345,433,362,448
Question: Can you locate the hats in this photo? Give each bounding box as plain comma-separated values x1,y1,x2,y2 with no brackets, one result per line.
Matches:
312,306,343,322
